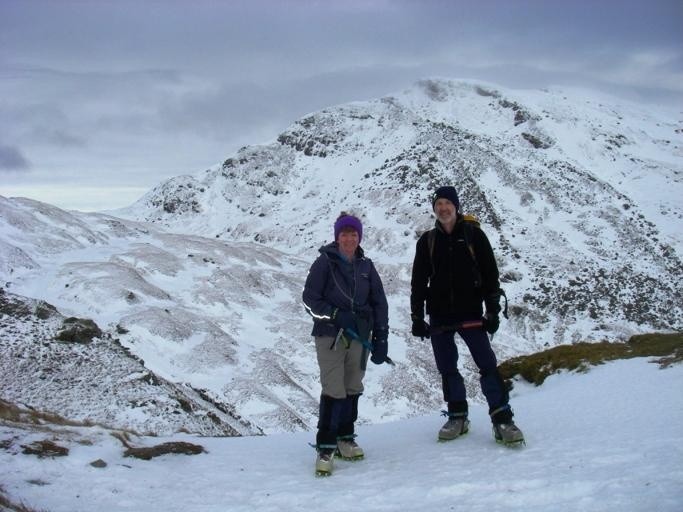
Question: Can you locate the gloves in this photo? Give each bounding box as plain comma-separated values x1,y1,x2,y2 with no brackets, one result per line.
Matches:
371,333,388,364
481,313,500,334
412,321,431,340
334,312,357,330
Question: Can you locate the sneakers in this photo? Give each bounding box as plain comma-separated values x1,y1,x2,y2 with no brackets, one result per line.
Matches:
336,436,364,461
315,446,336,477
493,423,524,446
439,419,468,440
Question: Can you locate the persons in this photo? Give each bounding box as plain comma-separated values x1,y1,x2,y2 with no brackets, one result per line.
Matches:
410,187,523,447
302,210,387,476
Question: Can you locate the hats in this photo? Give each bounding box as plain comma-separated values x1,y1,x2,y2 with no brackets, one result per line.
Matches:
334,216,362,243
432,187,459,211
